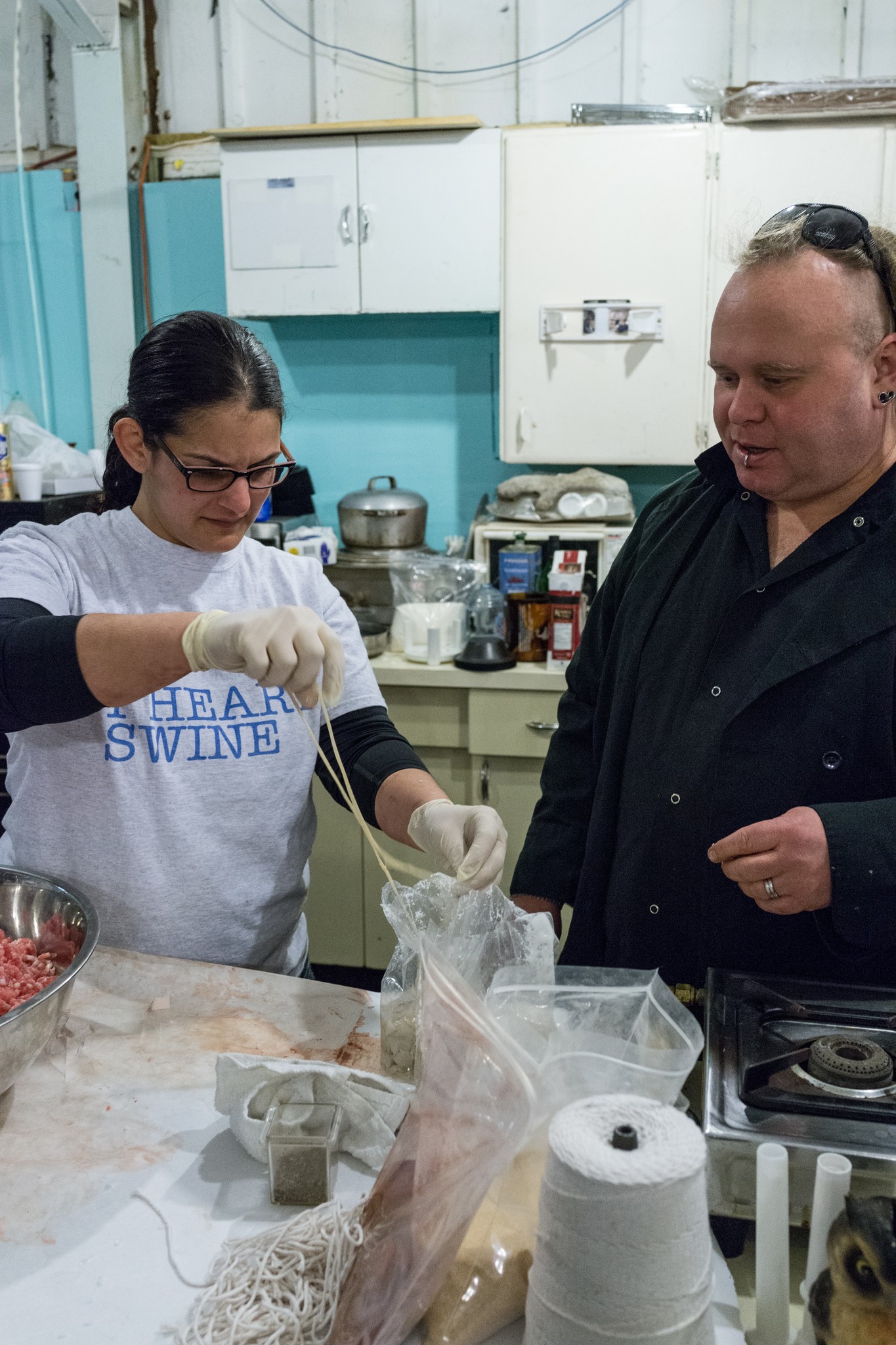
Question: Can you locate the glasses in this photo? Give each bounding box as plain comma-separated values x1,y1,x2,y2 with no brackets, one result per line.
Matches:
752,203,896,336
150,433,296,492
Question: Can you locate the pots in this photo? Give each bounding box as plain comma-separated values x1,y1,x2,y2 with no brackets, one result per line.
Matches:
336,476,427,547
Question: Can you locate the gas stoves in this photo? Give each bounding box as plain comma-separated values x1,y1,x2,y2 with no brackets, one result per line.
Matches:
681,968,896,1259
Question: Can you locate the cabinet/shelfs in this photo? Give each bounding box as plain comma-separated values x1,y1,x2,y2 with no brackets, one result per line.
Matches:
215,124,505,320
498,115,896,473
465,690,582,959
296,683,472,976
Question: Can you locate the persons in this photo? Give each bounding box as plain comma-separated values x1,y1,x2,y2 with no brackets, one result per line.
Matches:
518,201,893,983
0,311,511,979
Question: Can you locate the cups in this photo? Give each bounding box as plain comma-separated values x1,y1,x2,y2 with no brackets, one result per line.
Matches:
265,1101,344,1207
11,464,42,501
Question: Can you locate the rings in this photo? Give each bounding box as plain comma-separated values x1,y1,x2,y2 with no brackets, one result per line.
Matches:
763,878,779,899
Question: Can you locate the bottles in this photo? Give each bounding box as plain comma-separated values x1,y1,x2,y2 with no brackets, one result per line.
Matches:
0,423,14,502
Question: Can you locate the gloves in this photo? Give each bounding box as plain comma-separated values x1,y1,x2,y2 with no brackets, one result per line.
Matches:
407,798,509,893
181,606,344,709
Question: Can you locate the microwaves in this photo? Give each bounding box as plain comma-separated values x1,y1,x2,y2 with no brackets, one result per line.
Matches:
474,521,634,613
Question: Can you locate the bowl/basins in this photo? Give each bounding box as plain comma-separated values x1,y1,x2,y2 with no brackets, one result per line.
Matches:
357,620,387,659
0,864,102,1095
